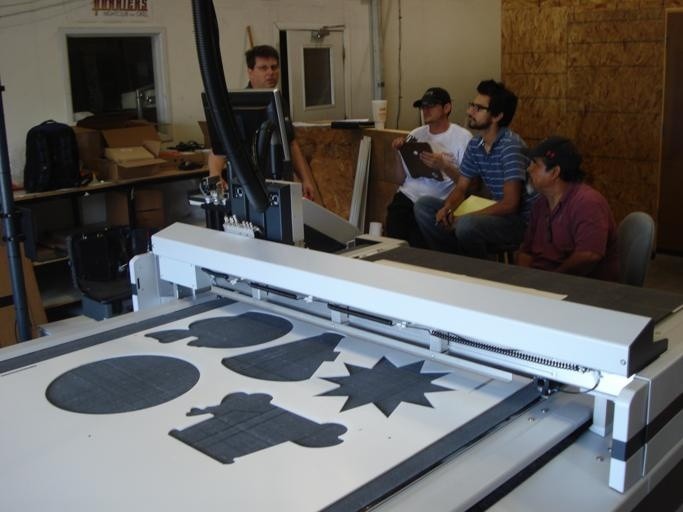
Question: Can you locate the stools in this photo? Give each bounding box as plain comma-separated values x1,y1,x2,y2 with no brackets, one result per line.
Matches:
78,275,134,320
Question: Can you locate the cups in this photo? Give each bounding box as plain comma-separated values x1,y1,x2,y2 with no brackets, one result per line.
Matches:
371,99,388,129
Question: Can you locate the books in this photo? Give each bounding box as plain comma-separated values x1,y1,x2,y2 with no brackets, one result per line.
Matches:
396,142,443,182
328,120,376,130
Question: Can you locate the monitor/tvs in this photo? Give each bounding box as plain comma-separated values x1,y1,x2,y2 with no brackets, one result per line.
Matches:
200,89,292,162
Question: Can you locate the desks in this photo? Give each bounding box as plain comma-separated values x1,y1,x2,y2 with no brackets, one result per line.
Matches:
10,159,230,331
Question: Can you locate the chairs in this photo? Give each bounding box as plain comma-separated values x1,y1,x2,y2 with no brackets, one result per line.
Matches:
487,210,656,286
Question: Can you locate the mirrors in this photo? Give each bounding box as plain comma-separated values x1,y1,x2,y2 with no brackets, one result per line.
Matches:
301,44,336,111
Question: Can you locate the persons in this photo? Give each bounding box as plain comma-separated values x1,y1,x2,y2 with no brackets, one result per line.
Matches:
411,78,543,257
384,87,476,257
510,134,621,287
205,43,317,214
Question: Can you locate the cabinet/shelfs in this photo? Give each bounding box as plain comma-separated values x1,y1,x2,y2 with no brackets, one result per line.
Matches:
293,117,411,232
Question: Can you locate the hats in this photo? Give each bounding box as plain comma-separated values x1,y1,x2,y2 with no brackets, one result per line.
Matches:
413,87,451,107
535,136,583,168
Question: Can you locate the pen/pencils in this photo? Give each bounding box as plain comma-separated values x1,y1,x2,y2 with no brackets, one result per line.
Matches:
434,208,452,227
405,135,411,141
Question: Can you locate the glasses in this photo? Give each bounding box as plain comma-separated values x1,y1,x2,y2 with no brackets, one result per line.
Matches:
468,103,490,114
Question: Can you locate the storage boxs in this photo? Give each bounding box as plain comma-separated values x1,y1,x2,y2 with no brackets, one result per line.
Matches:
69,119,168,182
105,187,165,229
